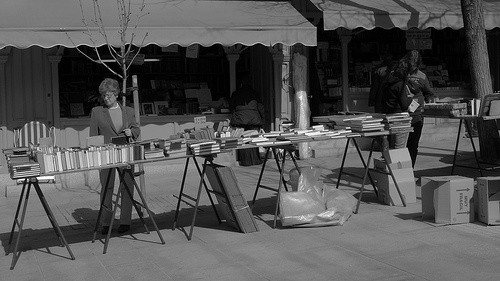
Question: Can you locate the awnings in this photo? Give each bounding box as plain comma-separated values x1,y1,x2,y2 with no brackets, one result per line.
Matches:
310,0,500,31
1,0,318,50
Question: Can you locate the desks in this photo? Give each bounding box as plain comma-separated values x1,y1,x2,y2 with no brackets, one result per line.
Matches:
1,116,500,270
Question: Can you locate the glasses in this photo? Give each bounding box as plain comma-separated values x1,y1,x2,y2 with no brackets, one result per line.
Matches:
100,92,115,99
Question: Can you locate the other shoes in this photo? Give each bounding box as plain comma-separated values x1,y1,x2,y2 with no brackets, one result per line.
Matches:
117,225,131,233
101,226,109,236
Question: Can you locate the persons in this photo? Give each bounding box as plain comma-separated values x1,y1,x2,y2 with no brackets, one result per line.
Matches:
229,78,266,166
370,57,409,158
88,78,141,234
398,49,434,175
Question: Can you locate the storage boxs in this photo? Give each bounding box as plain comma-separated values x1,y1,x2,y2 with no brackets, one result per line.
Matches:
373,147,500,226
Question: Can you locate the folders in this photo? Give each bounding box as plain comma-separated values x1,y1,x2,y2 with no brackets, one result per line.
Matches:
5,147,42,181
188,139,221,157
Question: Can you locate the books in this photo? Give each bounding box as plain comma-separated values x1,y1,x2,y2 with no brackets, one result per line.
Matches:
2,113,414,179
180,78,232,112
321,72,499,117
